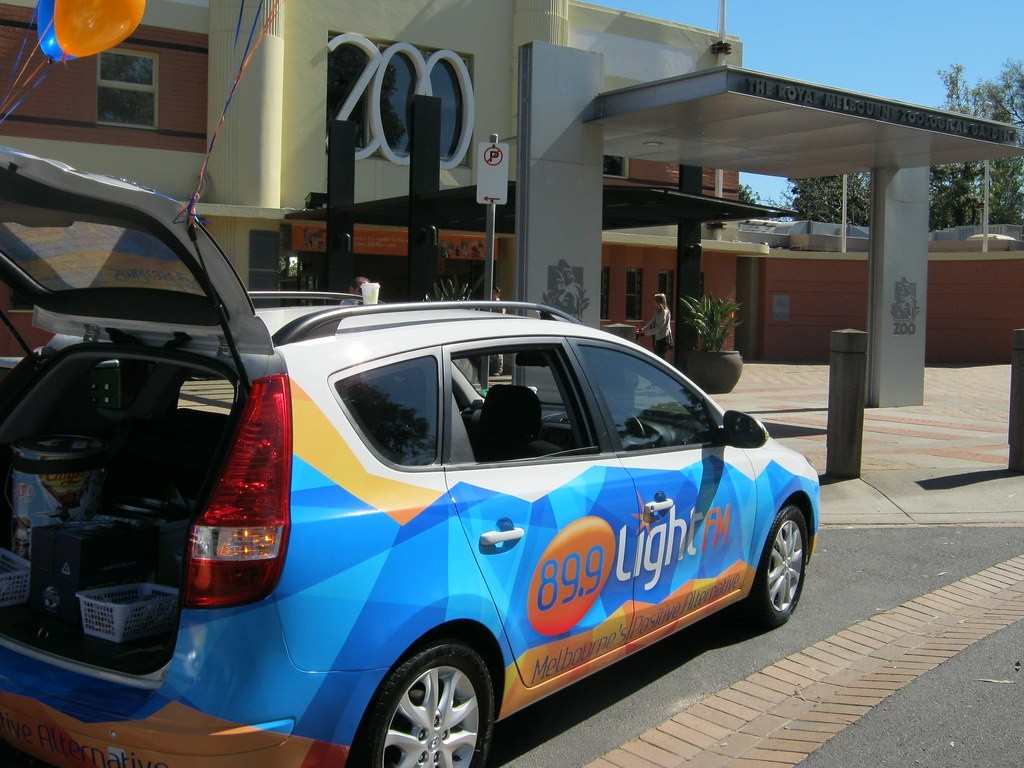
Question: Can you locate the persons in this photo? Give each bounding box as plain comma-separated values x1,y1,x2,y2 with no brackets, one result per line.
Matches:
493,287,505,377
341,277,369,304
641,293,670,360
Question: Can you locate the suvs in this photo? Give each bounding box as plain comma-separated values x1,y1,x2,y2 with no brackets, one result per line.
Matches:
0,148,822,768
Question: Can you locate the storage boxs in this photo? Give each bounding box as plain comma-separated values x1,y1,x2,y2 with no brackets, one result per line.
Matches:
159,518,190,586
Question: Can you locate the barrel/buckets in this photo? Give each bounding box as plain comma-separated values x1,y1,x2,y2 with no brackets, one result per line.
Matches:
3,432,114,562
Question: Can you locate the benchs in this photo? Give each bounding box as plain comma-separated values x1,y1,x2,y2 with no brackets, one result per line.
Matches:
141,409,229,498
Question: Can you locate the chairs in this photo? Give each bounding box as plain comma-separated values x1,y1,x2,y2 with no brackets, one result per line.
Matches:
477,385,562,458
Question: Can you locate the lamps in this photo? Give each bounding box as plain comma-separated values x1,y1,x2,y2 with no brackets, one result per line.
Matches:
686,244,701,262
304,193,328,209
418,225,438,248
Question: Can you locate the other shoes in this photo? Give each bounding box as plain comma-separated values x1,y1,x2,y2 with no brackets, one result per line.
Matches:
493,372,503,376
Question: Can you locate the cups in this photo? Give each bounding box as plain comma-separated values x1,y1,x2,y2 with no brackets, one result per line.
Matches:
360,282,381,306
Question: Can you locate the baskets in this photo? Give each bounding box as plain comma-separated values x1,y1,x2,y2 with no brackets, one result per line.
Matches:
0,548,31,607
74,583,180,643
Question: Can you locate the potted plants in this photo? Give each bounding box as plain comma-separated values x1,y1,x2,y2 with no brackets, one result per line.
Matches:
679,291,744,394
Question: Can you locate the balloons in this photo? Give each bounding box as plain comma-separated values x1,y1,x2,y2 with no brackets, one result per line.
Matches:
37,0,78,64
55,0,148,62
